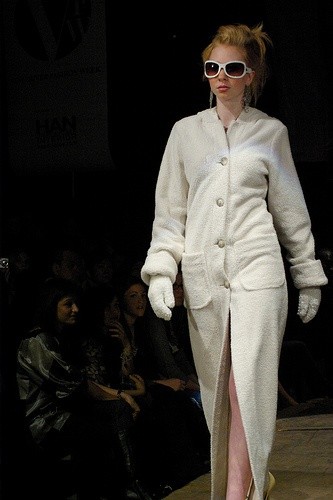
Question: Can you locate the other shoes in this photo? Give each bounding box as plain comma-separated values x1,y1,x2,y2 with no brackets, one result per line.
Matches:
265,472,276,500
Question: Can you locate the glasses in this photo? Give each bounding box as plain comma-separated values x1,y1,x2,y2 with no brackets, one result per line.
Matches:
173,281,183,290
204,60,252,79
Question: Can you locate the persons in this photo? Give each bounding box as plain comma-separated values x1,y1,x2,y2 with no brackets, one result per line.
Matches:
13,254,302,500
140,19,326,500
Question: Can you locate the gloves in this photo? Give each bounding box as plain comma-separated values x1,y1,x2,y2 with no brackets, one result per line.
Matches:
297,286,321,323
148,274,175,321
